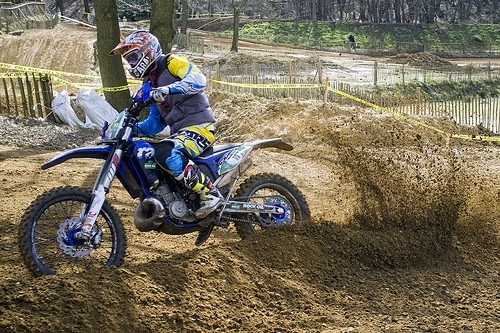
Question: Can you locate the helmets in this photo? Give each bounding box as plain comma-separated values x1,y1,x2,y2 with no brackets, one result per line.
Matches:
107,30,163,80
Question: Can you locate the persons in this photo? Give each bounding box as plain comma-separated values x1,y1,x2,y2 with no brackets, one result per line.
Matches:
101,31,225,218
349,35,355,48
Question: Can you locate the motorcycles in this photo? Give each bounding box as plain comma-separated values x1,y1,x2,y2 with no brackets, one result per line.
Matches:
18,81,311,278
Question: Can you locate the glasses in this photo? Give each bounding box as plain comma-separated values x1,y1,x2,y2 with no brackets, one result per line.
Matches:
124,41,152,68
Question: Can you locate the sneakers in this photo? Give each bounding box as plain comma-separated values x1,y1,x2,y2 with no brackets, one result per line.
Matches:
194,222,216,247
194,188,225,219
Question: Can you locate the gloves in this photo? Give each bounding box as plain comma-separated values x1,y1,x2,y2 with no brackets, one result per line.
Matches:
150,85,170,106
